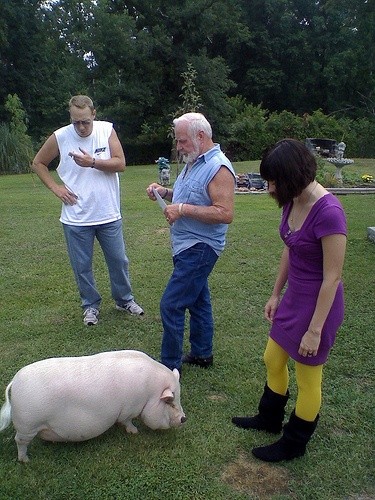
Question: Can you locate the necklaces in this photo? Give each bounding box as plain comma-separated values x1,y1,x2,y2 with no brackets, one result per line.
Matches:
290,182,317,230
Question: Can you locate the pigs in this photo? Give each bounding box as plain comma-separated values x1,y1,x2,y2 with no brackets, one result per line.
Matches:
0,350,190,463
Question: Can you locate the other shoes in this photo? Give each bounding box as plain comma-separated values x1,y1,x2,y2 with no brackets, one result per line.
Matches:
183,352,213,369
172,368,182,379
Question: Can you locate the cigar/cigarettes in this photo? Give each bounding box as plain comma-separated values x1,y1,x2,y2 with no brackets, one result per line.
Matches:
71,151,74,158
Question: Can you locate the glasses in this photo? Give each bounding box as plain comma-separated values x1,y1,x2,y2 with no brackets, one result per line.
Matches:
70,112,93,124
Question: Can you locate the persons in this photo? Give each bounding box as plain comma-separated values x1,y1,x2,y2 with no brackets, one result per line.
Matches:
33,95,145,326
147,113,237,375
233,139,348,461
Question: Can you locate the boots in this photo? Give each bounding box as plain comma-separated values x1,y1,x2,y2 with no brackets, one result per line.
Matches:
232,381,289,435
252,408,319,462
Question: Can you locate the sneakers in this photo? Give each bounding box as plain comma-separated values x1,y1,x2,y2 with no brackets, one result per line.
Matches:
116,300,144,316
83,308,99,326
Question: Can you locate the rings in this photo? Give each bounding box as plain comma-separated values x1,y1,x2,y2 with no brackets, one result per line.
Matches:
166,217,169,221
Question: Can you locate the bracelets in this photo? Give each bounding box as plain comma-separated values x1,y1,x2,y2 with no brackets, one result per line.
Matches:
161,188,168,199
92,158,95,169
178,203,183,216
